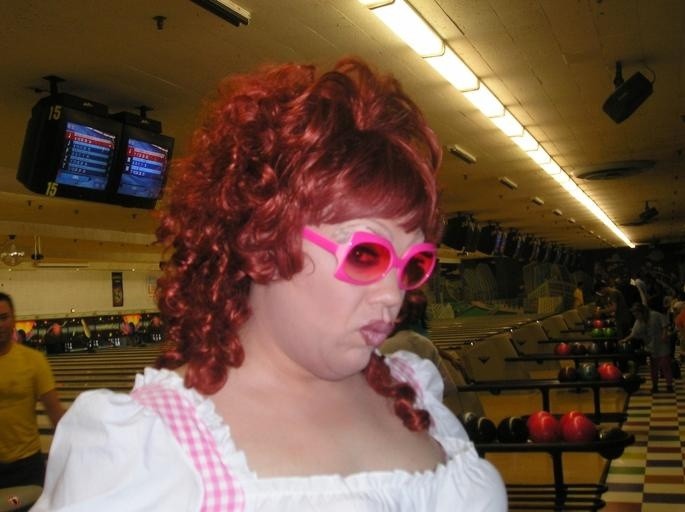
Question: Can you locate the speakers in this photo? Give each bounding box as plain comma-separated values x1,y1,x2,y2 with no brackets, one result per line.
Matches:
602,72,653,124
639,207,659,220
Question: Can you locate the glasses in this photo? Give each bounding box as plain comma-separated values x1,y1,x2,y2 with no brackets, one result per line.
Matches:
301,226,438,290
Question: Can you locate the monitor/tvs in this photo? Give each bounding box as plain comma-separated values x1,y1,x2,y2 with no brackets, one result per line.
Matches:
16,93,124,203
105,111,175,209
442,216,582,269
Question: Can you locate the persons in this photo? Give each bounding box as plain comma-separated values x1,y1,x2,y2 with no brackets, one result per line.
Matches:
16,330,27,344
26,54,509,512
572,279,584,306
592,262,685,392
1,291,73,486
